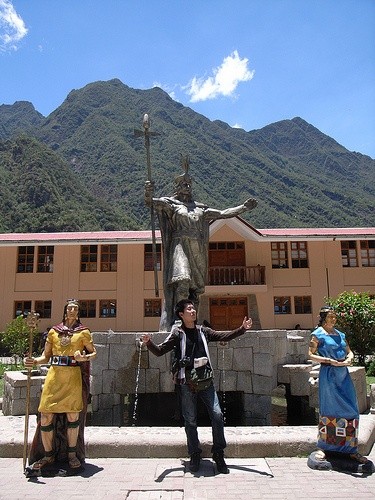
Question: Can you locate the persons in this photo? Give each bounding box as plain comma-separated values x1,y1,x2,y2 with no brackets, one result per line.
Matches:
24,300,96,469
309,307,366,462
139,299,252,475
145,155,258,332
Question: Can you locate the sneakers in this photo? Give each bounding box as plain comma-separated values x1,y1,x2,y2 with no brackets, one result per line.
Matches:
213,450,229,474
190,450,201,471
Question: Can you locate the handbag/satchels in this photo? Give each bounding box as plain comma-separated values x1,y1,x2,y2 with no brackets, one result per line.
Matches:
187,365,213,391
